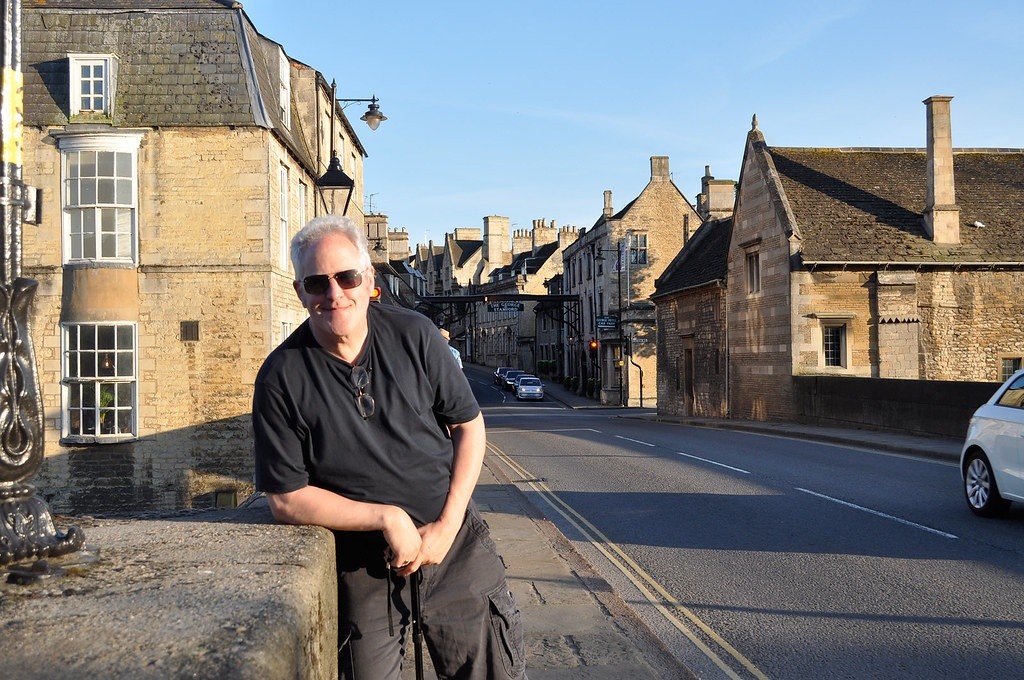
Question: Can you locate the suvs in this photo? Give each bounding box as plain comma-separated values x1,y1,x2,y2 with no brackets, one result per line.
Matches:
960,369,1024,514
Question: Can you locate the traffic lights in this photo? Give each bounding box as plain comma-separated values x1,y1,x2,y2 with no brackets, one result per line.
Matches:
625,335,631,355
371,285,382,305
589,342,597,360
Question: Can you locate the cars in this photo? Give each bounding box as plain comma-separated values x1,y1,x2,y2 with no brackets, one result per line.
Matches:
515,378,546,401
503,371,526,391
511,375,536,394
492,367,513,385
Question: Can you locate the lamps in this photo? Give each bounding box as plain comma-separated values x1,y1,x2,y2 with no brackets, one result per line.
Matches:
368,237,387,256
336,94,388,130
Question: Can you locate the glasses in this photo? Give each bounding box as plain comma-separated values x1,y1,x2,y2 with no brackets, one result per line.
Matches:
299,267,367,295
352,364,375,420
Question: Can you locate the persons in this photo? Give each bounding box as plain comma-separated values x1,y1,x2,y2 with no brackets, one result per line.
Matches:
439,327,463,369
253,215,529,680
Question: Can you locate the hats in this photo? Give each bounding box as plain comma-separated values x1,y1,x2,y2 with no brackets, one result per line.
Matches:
440,328,450,340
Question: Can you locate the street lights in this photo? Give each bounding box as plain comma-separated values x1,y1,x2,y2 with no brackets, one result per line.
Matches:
317,77,387,217
597,243,624,406
506,326,511,366
